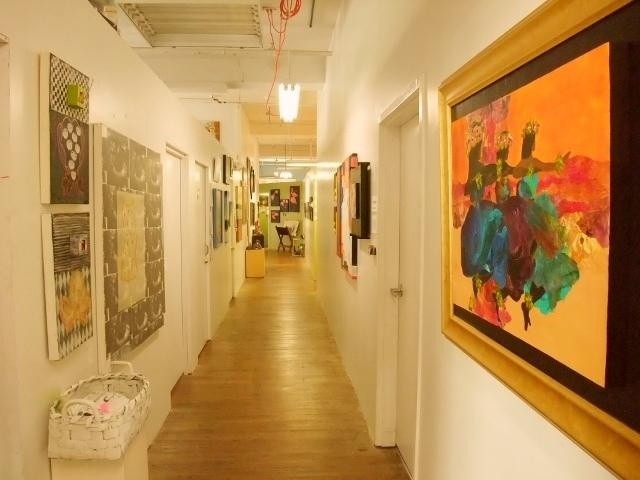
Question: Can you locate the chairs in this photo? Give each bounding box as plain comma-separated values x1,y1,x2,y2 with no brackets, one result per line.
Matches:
275,221,300,252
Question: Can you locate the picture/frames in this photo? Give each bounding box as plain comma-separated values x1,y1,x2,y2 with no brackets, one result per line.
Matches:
440,2,639,480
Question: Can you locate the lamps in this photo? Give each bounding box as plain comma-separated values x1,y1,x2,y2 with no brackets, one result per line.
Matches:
277,84,300,125
